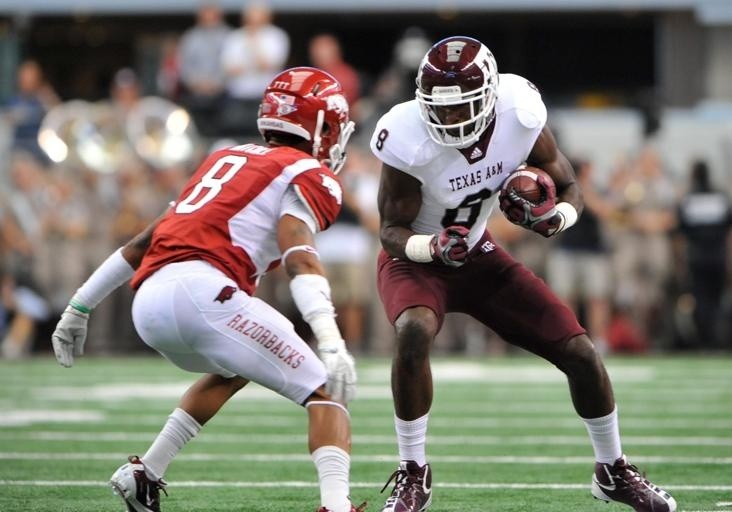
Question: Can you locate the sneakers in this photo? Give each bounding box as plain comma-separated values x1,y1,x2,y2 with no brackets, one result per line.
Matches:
110,455,168,512
379,458,432,512
591,454,677,512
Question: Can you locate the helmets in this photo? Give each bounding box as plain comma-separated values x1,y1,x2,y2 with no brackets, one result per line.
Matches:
416,36,498,151
255,67,356,174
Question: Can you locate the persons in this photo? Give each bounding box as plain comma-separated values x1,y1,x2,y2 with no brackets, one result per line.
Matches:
368,34,678,511
0,0,732,358
52,65,363,512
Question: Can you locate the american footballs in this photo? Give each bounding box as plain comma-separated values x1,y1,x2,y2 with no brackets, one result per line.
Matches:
501,165,556,205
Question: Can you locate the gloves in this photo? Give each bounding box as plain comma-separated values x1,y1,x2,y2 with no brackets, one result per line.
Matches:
429,226,470,269
318,337,358,408
499,174,562,239
51,305,89,368
